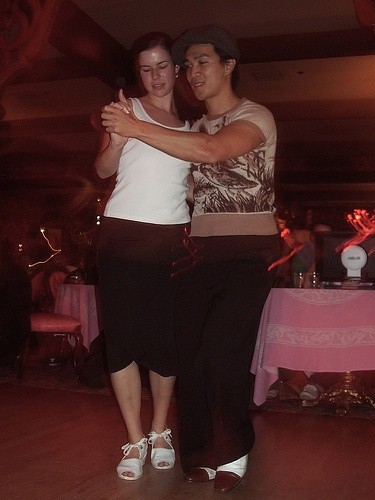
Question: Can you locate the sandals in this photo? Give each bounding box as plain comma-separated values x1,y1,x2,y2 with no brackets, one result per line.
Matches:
117,437,149,480
147,428,176,469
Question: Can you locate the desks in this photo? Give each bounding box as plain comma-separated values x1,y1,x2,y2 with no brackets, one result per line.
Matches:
248,287,375,418
53,285,102,366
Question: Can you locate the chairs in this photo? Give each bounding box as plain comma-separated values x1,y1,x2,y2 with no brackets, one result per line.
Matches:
10,265,83,379
44,263,70,307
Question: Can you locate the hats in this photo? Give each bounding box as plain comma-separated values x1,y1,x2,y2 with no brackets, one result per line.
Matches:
171,25,239,70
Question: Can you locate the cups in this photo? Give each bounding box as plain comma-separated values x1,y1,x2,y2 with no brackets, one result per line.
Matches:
293,271,304,288
310,271,320,288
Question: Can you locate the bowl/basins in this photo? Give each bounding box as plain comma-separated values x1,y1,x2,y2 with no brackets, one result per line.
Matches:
313,224,330,231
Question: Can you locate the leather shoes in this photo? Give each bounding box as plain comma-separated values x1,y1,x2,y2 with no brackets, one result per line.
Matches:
212,454,248,492
185,466,216,481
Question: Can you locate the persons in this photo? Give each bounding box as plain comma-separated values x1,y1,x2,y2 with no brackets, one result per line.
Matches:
0,203,321,366
93,34,196,480
102,33,280,494
